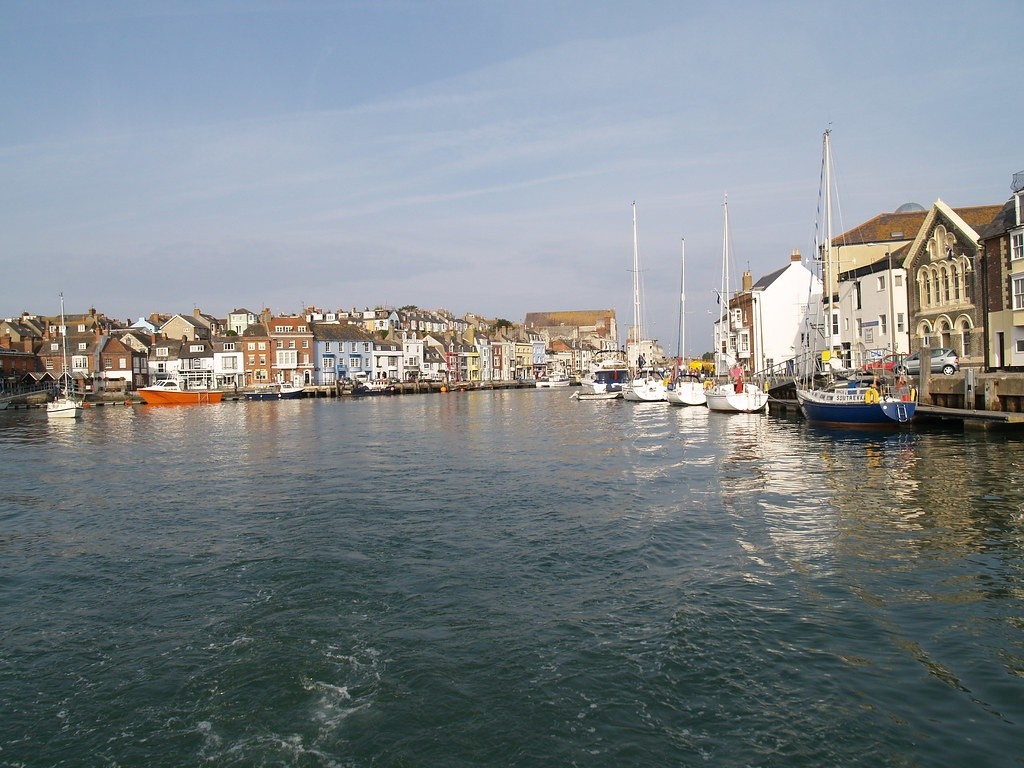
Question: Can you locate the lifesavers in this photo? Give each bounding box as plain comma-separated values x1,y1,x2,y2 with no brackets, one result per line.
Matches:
910,388,916,402
764,381,769,392
865,389,879,404
663,378,672,387
703,381,713,390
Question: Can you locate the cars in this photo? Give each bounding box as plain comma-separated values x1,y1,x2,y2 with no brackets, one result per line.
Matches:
862,354,913,372
894,347,960,376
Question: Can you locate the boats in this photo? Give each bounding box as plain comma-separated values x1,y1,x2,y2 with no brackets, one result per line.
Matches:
242,383,305,399
568,391,620,400
137,368,223,404
581,349,634,395
536,370,571,388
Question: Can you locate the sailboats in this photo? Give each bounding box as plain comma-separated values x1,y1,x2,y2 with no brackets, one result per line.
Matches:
704,192,770,413
46,291,84,418
795,120,916,428
621,200,666,402
666,237,709,406
